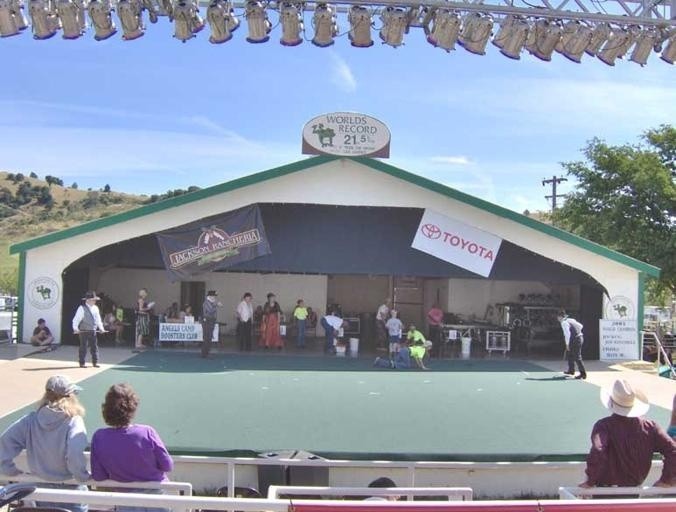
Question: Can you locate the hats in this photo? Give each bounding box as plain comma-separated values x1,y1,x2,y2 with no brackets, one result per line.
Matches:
207,290,218,297
600,376,651,418
44,375,82,397
80,290,102,301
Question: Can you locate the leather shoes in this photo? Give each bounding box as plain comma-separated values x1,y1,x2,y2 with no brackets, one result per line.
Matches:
576,374,587,379
563,370,575,375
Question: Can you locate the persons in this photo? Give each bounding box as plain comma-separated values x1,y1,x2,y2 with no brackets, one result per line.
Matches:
578,379,676,499
293,299,308,349
307,306,318,329
385,310,404,368
72,291,105,368
90,383,174,512
373,341,432,370
320,315,349,353
162,300,178,322
200,291,222,359
556,312,587,379
103,304,126,343
0,376,91,512
178,302,197,322
237,293,253,352
405,324,425,346
427,301,442,358
31,319,54,346
263,293,283,349
375,298,392,352
134,290,151,347
